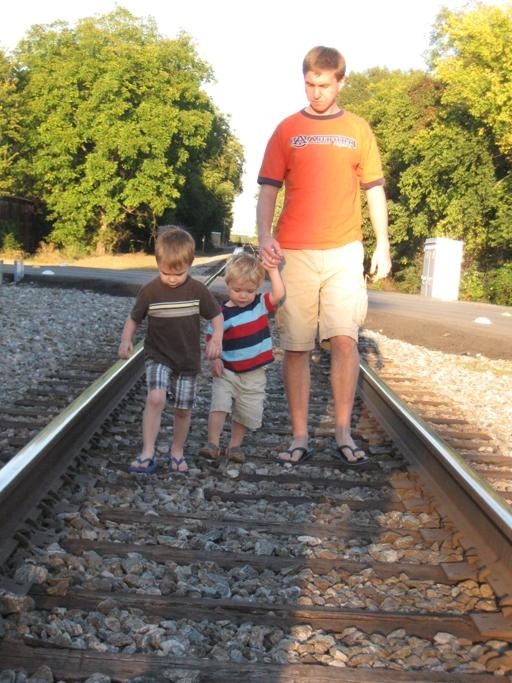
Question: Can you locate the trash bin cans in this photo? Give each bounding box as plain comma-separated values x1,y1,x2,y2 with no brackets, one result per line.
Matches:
420,237,463,300
211,232,221,248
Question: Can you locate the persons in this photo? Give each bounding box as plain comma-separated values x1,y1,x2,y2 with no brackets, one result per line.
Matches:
256,44,394,466
117,225,225,474
197,251,287,463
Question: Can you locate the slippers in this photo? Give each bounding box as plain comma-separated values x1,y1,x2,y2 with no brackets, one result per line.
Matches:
337,444,369,465
129,453,158,473
168,449,188,478
274,445,315,465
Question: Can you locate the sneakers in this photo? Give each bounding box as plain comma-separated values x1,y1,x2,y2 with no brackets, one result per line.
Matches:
225,446,246,463
200,443,221,460
